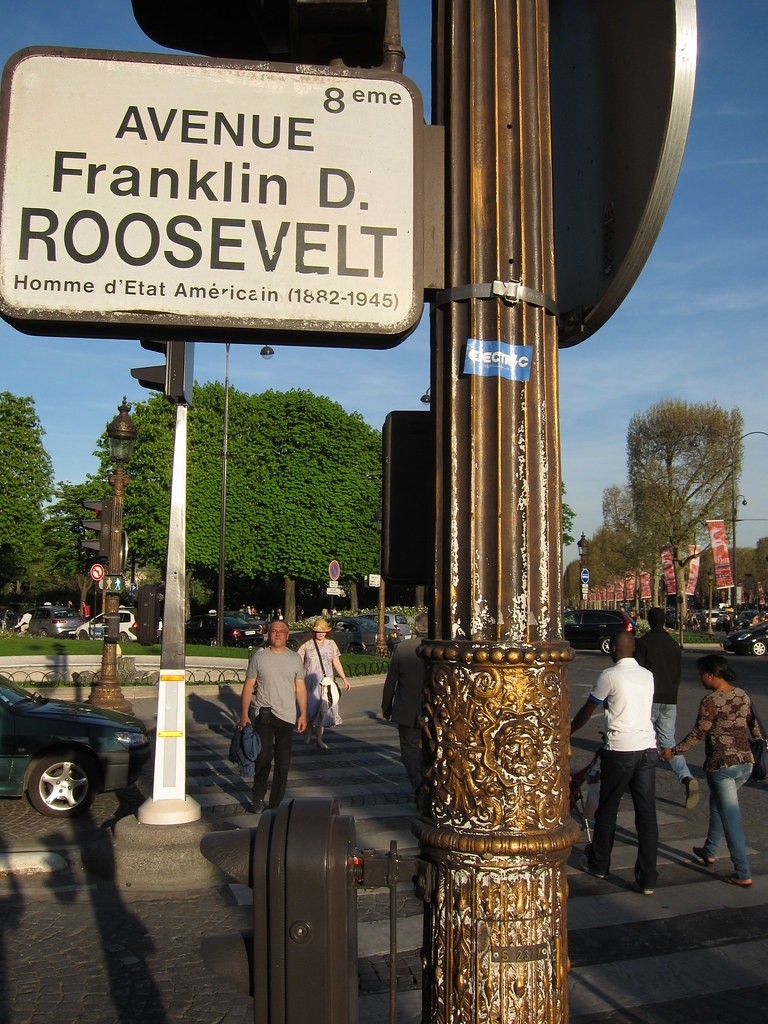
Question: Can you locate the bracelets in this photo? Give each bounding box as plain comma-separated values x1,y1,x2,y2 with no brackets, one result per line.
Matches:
342,676,346,679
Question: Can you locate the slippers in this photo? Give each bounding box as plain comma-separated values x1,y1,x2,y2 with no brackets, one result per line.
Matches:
692,846,715,866
722,874,746,888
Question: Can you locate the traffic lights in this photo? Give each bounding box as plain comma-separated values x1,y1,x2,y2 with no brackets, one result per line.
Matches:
81,500,112,559
129,585,160,645
130,338,193,405
197,796,360,1024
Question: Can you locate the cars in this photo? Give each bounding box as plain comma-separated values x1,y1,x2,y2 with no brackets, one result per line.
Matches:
563,608,636,655
284,617,402,656
724,620,768,656
1,672,152,817
186,612,265,648
1,604,84,639
75,606,163,643
357,613,411,640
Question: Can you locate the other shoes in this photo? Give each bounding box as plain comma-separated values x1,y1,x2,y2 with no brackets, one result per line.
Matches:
684,777,700,809
304,728,312,744
416,784,424,813
316,741,329,750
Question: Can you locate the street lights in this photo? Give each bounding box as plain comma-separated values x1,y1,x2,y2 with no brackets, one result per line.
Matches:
708,567,716,634
215,345,277,645
81,394,135,715
664,429,767,617
577,531,587,607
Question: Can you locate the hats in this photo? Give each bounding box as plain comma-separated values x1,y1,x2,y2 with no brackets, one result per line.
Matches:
310,618,332,632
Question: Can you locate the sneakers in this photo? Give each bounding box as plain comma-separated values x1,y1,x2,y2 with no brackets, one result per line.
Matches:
579,856,607,879
639,886,654,895
253,799,264,813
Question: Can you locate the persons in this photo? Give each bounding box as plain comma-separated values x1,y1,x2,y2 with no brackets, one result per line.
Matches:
382,615,427,807
81,601,91,620
570,632,658,895
67,601,74,608
724,615,732,636
239,604,337,622
661,654,764,887
573,605,585,610
635,607,700,809
620,604,637,621
297,620,350,748
15,609,32,632
752,613,767,626
494,719,545,776
239,620,307,814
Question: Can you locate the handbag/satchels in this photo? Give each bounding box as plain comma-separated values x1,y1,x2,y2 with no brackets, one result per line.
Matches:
747,736,768,781
582,756,601,818
320,677,342,711
